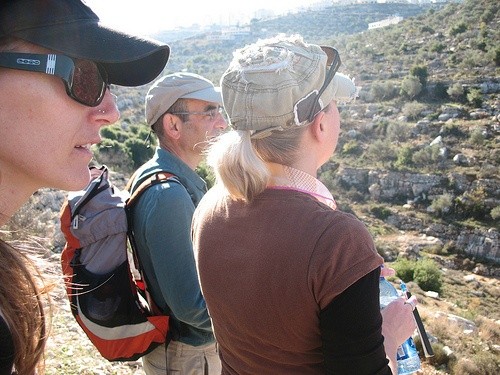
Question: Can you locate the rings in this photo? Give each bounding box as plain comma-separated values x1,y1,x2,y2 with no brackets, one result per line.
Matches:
405,299,416,310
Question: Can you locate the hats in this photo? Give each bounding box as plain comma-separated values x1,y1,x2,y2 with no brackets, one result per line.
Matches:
0,0,170,86
221,33,358,129
145,72,222,126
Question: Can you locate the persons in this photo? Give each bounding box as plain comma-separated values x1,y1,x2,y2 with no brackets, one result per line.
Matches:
1,0,120,375
123,71,227,375
190,33,418,375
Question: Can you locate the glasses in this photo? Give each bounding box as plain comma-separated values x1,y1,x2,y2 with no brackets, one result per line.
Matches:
0,51,110,107
308,45,342,122
171,106,224,121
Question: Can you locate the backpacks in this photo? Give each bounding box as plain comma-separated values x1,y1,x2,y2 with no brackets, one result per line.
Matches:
60,164,182,362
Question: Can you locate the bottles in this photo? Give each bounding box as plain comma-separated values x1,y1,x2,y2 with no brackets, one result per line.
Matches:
377,266,421,375
